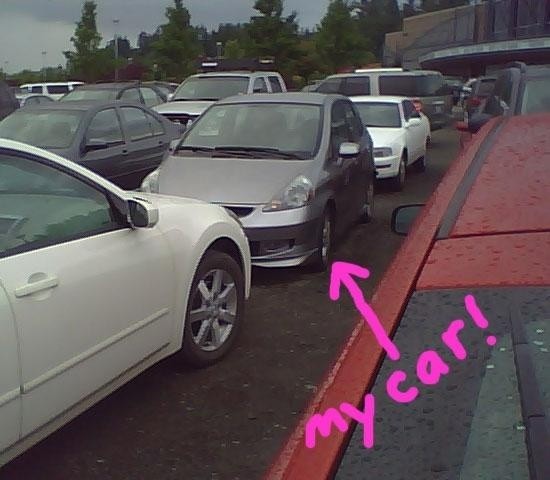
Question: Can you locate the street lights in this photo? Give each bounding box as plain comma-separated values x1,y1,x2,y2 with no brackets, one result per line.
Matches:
111,18,120,78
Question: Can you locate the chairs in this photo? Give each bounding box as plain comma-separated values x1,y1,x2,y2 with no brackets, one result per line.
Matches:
48,121,72,140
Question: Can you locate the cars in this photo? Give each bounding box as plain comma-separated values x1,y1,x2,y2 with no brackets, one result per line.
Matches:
0,60,549,479
